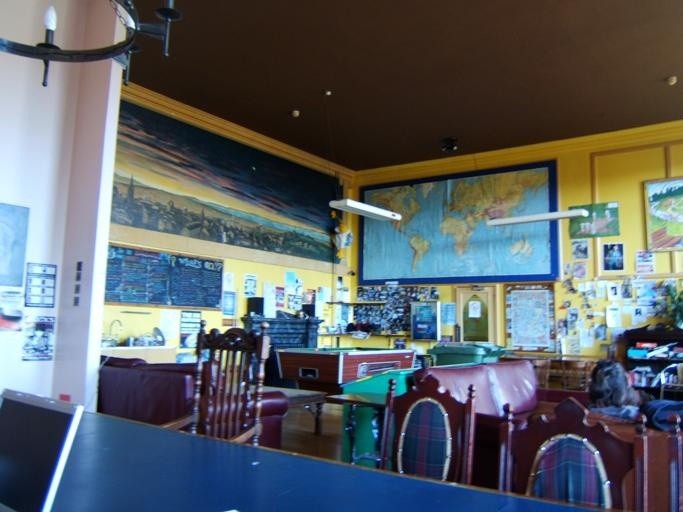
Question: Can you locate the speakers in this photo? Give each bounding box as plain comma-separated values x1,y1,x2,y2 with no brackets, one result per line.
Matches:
302,304,315,317
247,297,263,315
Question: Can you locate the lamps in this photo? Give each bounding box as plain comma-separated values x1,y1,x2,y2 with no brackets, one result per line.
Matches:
37,4,61,89
328,194,403,225
484,205,590,225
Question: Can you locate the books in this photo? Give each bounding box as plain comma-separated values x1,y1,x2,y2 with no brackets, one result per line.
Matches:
180,308,202,346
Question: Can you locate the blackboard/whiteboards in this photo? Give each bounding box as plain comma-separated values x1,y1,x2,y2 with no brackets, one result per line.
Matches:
105,240,224,310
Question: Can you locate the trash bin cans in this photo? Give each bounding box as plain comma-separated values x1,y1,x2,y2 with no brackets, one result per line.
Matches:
427,342,507,366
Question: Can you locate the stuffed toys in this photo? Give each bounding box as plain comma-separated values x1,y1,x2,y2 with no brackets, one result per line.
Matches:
588,359,656,408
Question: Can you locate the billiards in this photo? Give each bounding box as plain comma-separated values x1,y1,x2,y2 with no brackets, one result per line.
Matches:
313,346,317,352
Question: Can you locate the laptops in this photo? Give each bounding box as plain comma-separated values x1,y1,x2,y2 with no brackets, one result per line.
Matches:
0,388,85,512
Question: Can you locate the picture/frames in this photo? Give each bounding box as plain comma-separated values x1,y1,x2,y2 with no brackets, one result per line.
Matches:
357,156,564,284
109,86,352,277
641,176,683,253
